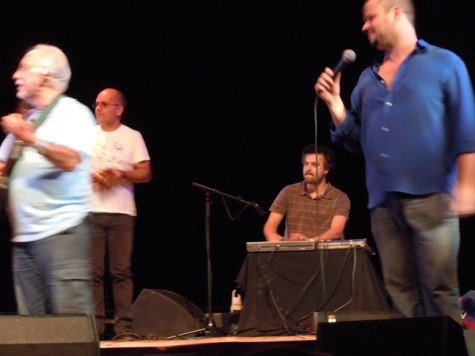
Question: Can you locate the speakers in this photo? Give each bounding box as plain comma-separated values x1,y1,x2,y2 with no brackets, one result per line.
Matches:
129,289,206,337
0,313,100,356
316,315,469,356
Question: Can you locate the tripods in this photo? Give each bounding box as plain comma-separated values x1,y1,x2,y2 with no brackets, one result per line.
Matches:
165,182,254,339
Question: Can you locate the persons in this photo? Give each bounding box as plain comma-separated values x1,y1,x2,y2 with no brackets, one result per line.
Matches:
0,44,96,314
315,0,475,326
263,145,350,242
86,88,151,341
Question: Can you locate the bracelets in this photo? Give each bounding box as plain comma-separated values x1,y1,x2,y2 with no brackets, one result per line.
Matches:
36,140,52,156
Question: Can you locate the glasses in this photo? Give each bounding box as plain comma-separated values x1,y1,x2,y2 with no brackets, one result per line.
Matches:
92,101,119,108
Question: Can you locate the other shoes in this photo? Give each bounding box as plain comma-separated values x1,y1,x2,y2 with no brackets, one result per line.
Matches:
118,331,137,341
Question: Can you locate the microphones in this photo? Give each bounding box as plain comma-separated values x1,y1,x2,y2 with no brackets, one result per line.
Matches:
331,49,357,78
253,202,266,216
